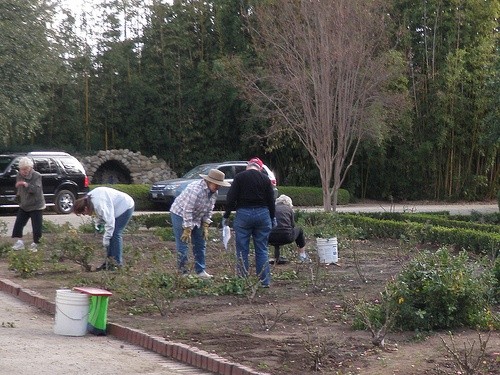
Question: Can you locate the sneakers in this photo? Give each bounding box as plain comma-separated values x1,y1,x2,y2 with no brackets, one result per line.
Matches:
194,270,213,279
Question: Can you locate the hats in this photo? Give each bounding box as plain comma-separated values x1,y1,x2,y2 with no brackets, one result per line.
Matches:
199,168,232,188
248,157,262,169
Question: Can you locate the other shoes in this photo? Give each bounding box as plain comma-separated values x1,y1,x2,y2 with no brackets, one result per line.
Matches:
12,240,25,250
297,254,312,264
96,262,110,271
28,243,39,252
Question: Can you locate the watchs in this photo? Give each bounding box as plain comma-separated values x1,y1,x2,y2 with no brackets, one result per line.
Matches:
23,182,26,185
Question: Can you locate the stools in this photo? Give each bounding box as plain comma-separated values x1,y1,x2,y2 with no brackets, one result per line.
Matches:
267,242,290,264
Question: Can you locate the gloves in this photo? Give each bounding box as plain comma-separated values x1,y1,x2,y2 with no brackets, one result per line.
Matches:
201,223,209,241
95,223,101,231
273,218,277,228
180,228,192,243
222,218,228,227
103,240,110,247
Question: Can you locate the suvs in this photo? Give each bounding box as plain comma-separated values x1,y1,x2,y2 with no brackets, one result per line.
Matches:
150,161,278,210
0,152,89,214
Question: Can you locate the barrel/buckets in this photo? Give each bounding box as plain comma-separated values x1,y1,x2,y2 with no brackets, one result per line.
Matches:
316,237,338,264
54,289,93,336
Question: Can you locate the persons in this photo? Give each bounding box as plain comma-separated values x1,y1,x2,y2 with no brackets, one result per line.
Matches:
267,194,312,264
8,157,47,252
72,186,135,270
170,168,232,279
221,158,278,288
